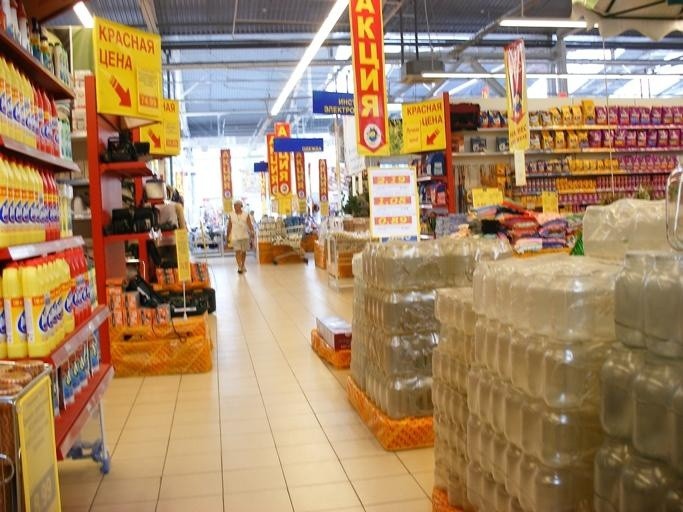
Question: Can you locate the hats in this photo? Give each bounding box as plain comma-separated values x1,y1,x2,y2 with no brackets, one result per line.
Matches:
233,200,242,206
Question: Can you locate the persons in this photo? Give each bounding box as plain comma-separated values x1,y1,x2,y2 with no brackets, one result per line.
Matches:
312,205,320,225
154,196,189,231
247,210,257,231
225,200,255,273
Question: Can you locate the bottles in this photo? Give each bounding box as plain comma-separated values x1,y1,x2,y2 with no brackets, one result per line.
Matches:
433,200,681,512
350,236,516,413
663,153,683,253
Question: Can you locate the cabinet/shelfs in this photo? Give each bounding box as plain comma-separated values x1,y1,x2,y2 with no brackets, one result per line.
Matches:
0,28,155,474
415,177,447,240
450,97,682,218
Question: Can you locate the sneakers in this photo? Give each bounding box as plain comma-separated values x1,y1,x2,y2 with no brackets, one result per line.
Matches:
238,268,242,273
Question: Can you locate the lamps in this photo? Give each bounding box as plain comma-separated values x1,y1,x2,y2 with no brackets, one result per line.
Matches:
270,0,349,119
498,0,600,29
